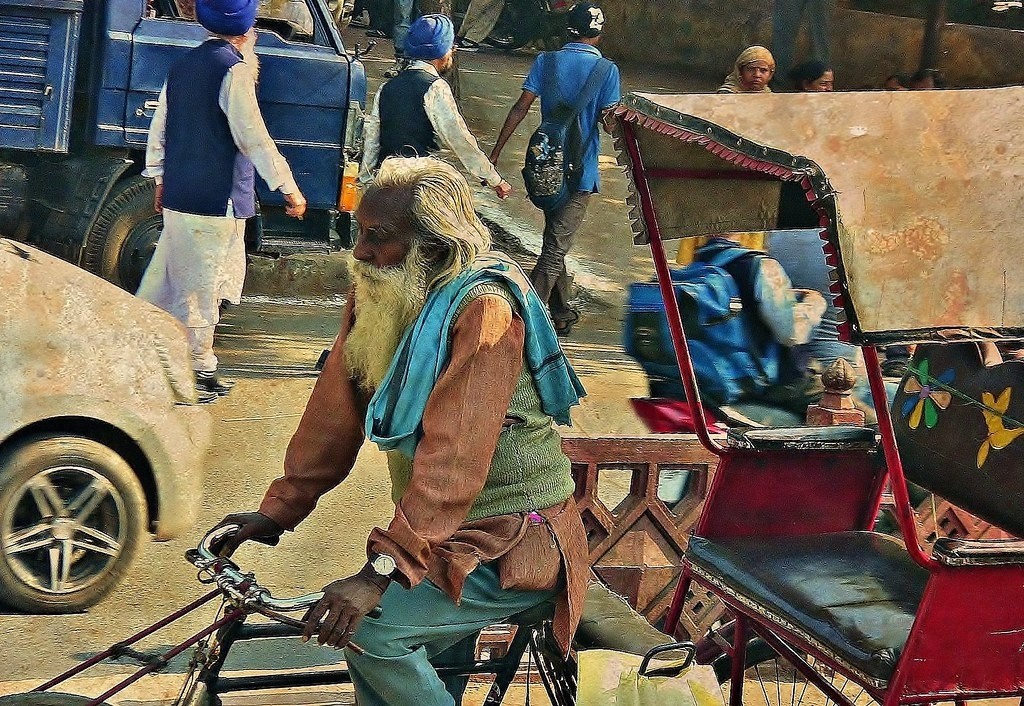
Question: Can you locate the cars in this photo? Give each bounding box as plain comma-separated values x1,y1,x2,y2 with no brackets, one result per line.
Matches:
0,239,213,616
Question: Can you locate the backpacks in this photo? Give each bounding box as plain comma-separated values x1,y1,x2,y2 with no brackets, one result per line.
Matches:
522,50,615,213
625,242,788,406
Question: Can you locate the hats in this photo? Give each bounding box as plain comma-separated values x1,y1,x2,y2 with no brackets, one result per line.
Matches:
404,14,454,59
567,2,604,37
195,0,255,35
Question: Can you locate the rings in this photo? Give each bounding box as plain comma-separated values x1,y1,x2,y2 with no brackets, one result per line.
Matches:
503,195,508,199
346,631,355,634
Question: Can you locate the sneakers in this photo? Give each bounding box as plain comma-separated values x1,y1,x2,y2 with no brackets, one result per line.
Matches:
196,391,218,403
195,371,234,394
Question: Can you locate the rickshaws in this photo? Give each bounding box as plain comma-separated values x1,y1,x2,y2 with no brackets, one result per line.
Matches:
3,94,1024,706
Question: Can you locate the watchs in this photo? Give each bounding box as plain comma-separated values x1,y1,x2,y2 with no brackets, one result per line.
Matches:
367,553,398,581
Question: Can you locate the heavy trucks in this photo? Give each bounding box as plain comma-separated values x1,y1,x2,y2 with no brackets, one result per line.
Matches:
0,0,378,294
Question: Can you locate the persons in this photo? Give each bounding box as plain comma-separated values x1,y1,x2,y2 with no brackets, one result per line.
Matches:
366,0,395,38
454,0,504,51
480,0,619,336
678,47,775,266
135,2,306,401
793,62,834,90
773,229,857,369
697,231,882,425
361,14,512,200
214,157,589,706
885,69,945,88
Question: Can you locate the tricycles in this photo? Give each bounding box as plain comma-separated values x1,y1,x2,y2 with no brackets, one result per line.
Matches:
0,84,1024,706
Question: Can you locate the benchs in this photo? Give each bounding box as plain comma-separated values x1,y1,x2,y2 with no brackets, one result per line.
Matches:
669,344,1024,706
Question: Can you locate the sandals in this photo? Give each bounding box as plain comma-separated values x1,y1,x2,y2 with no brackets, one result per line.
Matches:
553,309,580,336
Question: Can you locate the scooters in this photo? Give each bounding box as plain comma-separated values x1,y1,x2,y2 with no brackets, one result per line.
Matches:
447,0,551,50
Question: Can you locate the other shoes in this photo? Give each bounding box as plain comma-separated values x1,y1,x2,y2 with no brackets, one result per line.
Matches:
366,29,390,38
455,39,479,52
384,58,410,77
